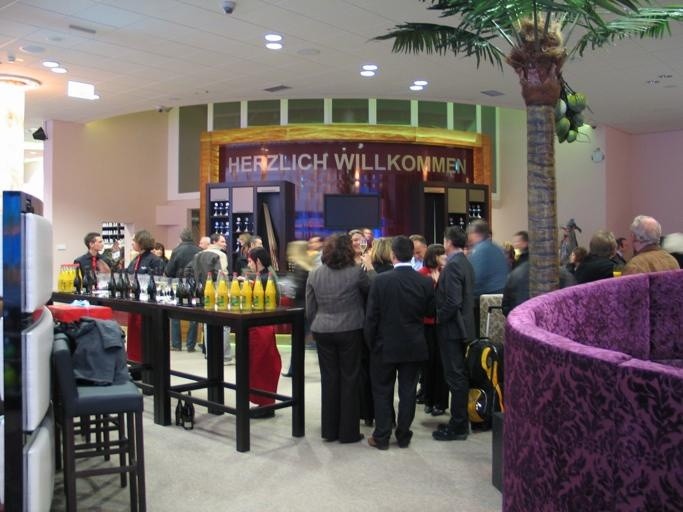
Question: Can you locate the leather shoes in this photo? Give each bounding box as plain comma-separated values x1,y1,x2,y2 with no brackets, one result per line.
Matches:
367,437,385,448
187,348,196,352
170,347,181,351
416,389,469,441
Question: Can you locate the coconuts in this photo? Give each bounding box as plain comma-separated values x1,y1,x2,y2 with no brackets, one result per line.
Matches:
554,92,586,143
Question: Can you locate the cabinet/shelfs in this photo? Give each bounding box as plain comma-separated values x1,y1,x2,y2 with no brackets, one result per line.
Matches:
206,180,296,281
419,180,489,250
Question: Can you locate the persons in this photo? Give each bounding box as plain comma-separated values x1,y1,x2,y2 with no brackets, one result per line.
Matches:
288,216,682,449
73,227,281,363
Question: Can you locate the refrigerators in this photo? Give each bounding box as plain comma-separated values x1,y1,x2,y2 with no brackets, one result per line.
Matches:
1,188,60,512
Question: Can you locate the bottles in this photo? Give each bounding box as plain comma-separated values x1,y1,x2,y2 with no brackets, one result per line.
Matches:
213,202,250,253
174,391,194,432
58,263,281,312
449,204,482,229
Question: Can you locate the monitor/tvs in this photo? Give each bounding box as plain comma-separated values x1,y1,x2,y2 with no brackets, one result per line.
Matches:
324,194,379,228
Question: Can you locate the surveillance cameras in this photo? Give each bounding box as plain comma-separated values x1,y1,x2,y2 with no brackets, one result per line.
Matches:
220,1,235,14
156,105,164,113
592,125,596,129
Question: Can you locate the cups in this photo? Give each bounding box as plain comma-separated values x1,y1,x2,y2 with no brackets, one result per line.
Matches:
612,264,624,277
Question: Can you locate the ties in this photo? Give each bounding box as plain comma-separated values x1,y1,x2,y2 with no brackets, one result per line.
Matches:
92,256,97,271
133,255,141,272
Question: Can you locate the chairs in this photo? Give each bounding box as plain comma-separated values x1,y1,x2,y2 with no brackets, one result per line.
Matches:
52,332,148,512
